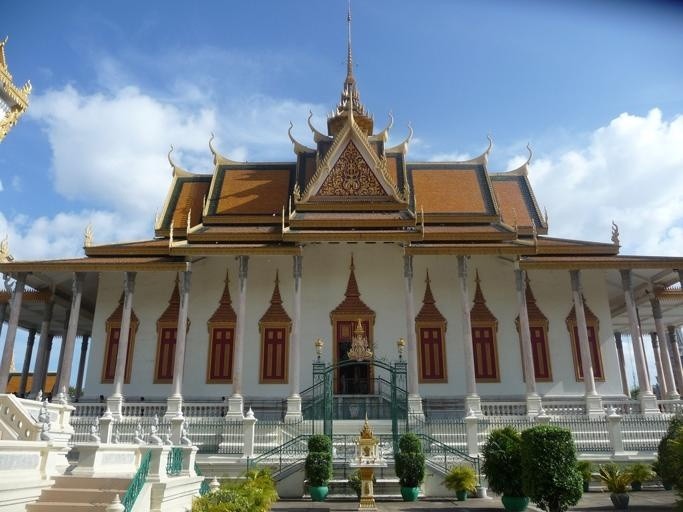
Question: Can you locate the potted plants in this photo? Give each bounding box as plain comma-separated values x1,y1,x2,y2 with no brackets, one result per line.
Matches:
348,470,377,499
440,464,479,500
304,451,333,500
578,459,675,511
479,426,534,512
394,452,426,501
